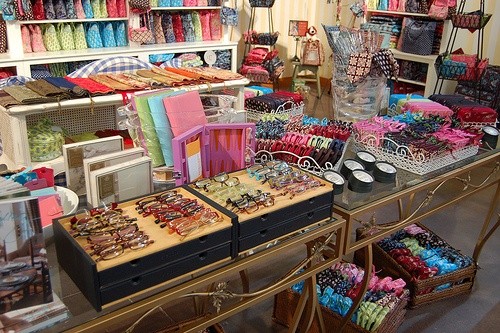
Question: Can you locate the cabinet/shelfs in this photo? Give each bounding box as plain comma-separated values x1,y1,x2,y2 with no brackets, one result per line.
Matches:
363,0,450,99
0,0,250,177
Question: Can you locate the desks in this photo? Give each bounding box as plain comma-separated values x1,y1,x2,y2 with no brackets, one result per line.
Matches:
0,213,346,333
333,143,500,333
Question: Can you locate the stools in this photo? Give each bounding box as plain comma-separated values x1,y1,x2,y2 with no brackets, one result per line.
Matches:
291,61,321,99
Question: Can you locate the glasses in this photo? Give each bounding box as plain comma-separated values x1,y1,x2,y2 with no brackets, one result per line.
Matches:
68,159,327,262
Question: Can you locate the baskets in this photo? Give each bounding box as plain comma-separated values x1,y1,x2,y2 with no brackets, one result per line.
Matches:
243,100,305,124
350,130,483,176
272,249,410,333
354,220,476,311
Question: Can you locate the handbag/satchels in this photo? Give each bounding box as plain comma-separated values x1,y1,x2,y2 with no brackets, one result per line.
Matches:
438,47,490,81
364,0,458,56
239,47,284,82
0,0,238,54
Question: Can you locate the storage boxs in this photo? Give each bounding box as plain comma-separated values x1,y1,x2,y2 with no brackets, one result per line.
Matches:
52,156,334,313
